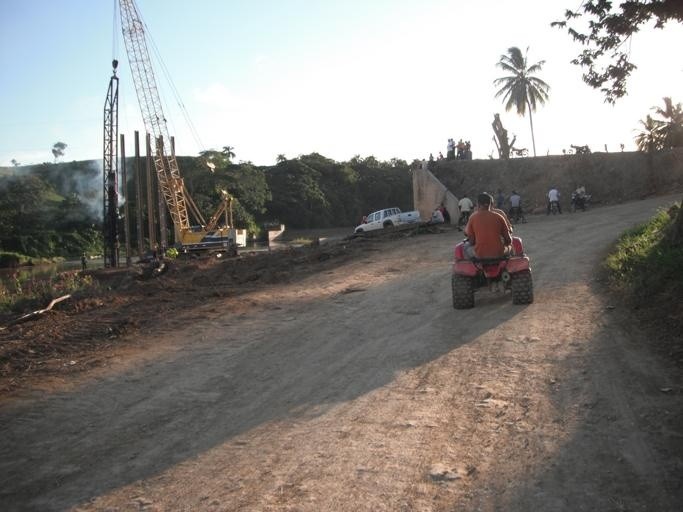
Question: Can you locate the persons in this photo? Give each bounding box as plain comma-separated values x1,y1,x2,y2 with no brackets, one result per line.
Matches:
546,185,562,216
468,193,512,235
411,138,471,169
458,193,476,220
496,190,505,211
361,216,367,225
575,184,586,212
428,208,444,225
80,253,87,271
508,190,527,224
436,204,444,214
463,191,512,258
442,208,449,223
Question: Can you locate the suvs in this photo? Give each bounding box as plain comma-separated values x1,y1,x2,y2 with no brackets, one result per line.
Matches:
450,237,536,309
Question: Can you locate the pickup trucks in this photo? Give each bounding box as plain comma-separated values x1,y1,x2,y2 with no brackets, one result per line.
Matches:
353,206,422,235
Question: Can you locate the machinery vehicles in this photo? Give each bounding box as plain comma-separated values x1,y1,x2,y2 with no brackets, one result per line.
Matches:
115,0,247,257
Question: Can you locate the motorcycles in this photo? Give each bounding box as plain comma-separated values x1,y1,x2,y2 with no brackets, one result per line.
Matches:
454,194,589,227
